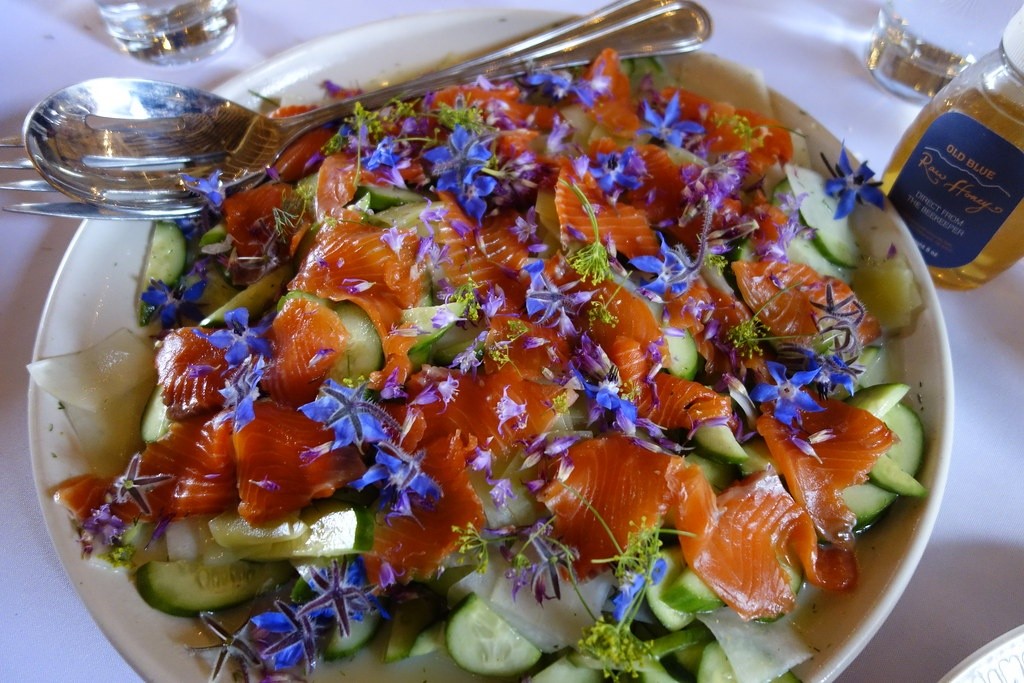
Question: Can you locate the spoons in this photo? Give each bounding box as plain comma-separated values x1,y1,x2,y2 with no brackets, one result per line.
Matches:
21,2,711,214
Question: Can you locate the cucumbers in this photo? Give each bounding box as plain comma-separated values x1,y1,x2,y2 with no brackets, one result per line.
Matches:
132,103,925,682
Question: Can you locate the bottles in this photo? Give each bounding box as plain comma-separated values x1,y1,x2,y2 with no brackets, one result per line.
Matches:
879,4,1023,292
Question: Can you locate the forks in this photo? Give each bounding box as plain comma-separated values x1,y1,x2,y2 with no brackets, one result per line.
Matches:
0,1,681,212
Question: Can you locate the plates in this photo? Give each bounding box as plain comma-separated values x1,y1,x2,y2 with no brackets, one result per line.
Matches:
24,10,952,683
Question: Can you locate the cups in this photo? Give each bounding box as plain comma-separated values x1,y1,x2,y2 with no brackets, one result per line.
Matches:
91,1,238,65
867,1,977,100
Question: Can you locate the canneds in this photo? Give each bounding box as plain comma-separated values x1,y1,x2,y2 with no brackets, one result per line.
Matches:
875,2,1023,295
